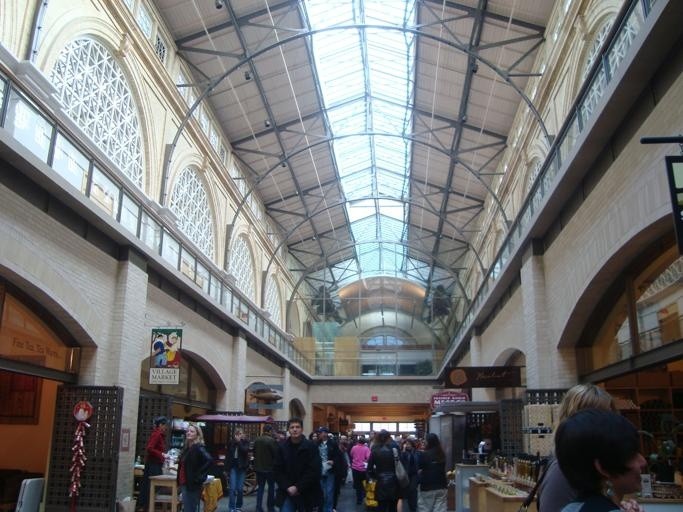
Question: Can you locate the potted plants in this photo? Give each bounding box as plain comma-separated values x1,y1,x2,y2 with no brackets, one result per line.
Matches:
633,412,682,484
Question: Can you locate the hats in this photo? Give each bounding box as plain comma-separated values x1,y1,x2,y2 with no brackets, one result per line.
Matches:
155,416,170,425
316,426,328,432
406,435,418,439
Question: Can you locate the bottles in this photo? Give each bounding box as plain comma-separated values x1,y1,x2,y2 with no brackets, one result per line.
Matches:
516,455,537,482
494,455,507,474
529,421,552,433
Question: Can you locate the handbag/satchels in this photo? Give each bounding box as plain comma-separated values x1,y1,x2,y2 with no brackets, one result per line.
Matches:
395,460,409,487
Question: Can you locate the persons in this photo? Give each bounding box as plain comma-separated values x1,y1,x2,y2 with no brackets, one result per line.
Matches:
176,422,213,512
251,418,503,511
222,426,250,512
134,416,171,512
537,382,619,512
554,408,649,512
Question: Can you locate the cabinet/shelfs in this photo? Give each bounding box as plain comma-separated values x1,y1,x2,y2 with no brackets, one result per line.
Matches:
147,473,183,512
